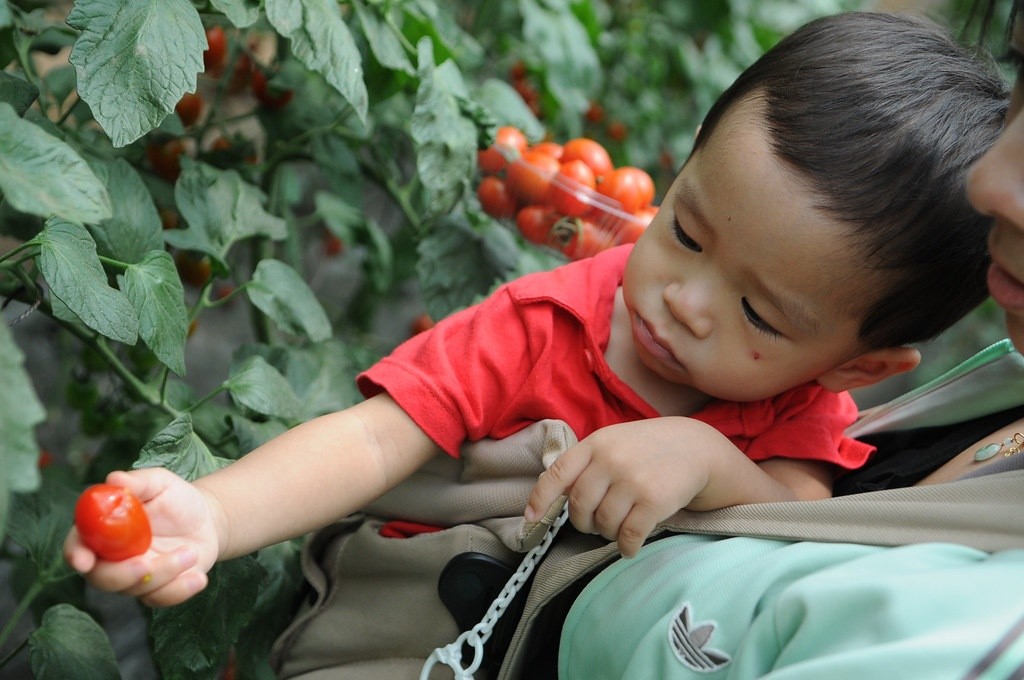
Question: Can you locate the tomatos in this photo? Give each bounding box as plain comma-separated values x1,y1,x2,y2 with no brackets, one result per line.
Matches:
141,30,435,340
74,483,151,562
475,61,663,261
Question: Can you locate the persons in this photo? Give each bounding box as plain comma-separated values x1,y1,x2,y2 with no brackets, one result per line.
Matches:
554,1,1024,680
61,10,1014,610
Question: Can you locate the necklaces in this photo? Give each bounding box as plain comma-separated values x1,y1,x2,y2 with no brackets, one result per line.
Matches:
973,432,1024,462
